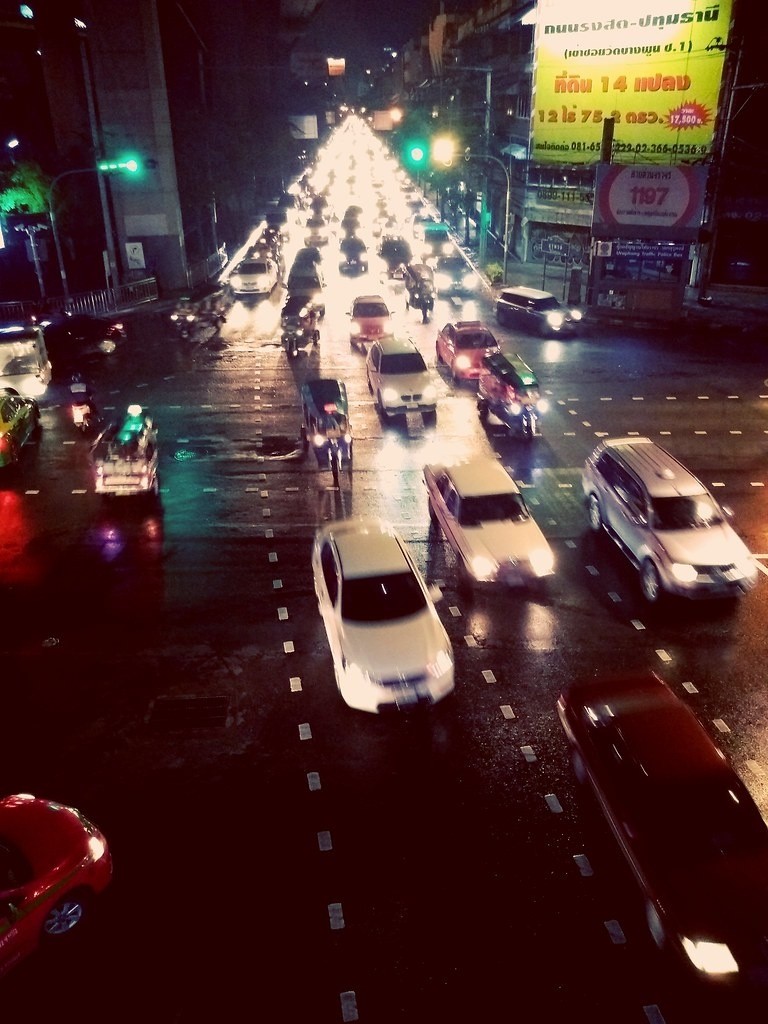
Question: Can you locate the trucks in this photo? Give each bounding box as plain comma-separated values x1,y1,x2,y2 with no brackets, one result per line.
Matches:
89,404,161,498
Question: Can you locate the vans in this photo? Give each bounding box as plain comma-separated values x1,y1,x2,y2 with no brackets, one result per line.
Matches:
0,326,52,398
496,285,575,337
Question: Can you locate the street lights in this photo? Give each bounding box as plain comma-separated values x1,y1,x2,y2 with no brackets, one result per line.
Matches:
7,138,46,303
433,152,510,285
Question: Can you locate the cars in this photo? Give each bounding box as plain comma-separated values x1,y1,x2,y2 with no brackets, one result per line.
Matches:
557,666,768,976
313,517,455,713
424,455,555,582
280,298,324,328
19,314,127,369
339,205,369,277
398,183,453,259
302,190,335,247
283,247,326,300
230,260,279,294
371,213,412,280
345,294,395,346
0,791,113,982
0,396,39,468
248,194,302,261
435,320,501,382
432,257,475,295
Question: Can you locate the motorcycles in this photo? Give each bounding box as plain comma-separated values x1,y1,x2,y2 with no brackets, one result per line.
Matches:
403,265,436,321
300,379,354,478
281,325,319,357
172,300,228,334
474,351,541,439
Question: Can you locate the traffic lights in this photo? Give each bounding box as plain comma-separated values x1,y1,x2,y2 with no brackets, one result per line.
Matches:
410,144,425,163
97,160,137,172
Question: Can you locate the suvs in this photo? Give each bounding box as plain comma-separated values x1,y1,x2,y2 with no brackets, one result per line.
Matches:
584,437,758,601
366,336,436,418
